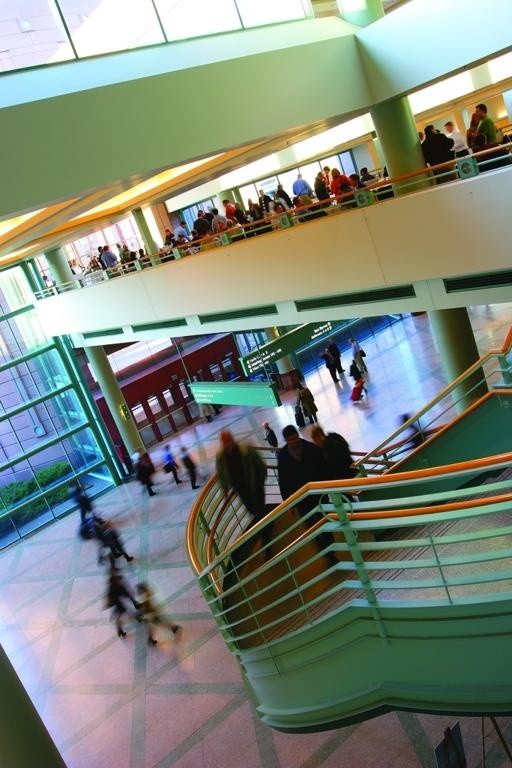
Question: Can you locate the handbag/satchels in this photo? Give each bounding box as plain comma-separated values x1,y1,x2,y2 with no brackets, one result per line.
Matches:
360,350,367,357
355,379,366,387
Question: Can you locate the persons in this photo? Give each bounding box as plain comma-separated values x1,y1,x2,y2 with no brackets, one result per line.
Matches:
235,175,326,237
263,421,278,456
321,338,368,398
70,482,134,571
399,413,425,447
199,403,221,423
314,167,393,209
418,103,503,181
69,243,153,286
216,428,273,557
104,567,180,645
163,199,235,258
278,424,358,567
134,443,200,496
296,384,318,425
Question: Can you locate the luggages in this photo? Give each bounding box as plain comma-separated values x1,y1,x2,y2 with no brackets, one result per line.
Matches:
295,406,305,427
351,387,362,401
